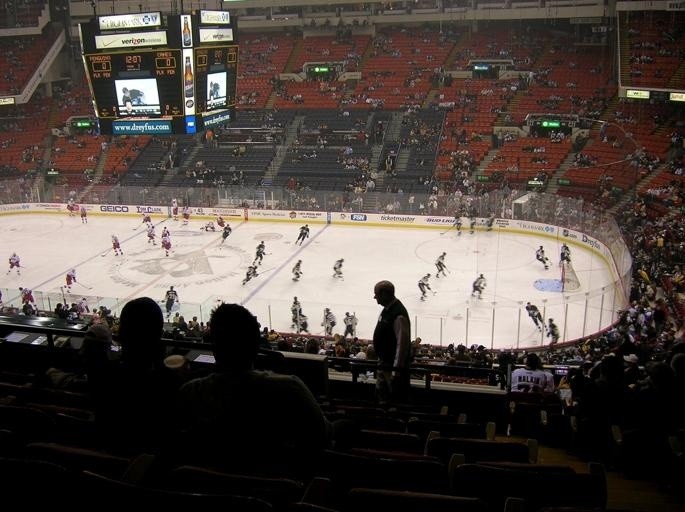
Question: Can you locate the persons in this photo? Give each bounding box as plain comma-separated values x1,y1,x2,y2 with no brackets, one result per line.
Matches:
418,252,487,301
592,236,684,281
111,224,233,256
536,243,572,269
2,281,684,512
242,224,345,285
1,1,685,236
5,252,21,275
63,269,77,293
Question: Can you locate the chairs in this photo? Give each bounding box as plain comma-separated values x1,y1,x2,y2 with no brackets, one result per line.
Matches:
0,36,685,512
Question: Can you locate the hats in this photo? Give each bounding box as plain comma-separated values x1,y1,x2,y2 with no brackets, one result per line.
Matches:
85,324,119,346
604,353,616,357
624,354,638,363
